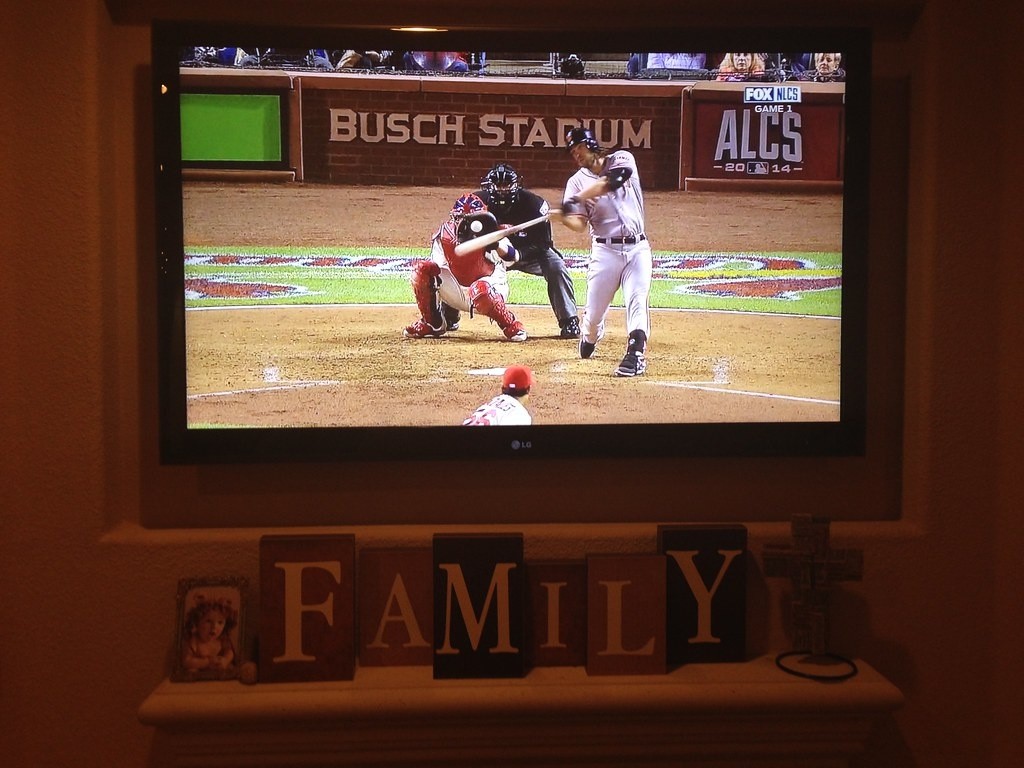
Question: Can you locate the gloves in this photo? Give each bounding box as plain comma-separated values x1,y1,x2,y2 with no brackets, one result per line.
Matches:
563,195,581,214
483,241,499,251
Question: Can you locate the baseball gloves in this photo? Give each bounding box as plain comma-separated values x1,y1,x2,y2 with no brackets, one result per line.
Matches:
456,211,499,253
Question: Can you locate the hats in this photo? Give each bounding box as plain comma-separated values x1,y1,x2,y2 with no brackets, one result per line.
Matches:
503,364,532,390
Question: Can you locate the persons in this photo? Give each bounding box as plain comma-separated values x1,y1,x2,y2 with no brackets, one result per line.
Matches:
402,127,651,425
188,45,844,80
182,593,237,675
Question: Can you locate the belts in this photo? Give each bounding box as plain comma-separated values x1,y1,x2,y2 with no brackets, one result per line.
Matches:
596,234,645,244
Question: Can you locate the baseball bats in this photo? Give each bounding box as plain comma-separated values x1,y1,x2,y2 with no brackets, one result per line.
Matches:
454,214,548,256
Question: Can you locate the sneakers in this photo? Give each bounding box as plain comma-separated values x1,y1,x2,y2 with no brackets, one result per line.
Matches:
615,339,647,378
579,336,596,359
560,317,580,339
511,328,528,342
403,315,448,338
446,316,460,330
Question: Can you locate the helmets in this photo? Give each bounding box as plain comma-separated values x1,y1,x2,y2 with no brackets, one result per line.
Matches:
480,163,523,214
565,128,597,151
450,192,487,218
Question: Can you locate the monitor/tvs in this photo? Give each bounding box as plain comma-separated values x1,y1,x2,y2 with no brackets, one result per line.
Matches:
151,19,872,457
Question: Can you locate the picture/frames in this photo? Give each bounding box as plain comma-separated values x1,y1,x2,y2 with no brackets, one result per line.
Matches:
174,577,249,683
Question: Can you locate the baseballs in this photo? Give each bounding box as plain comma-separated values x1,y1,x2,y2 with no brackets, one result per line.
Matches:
471,221,482,232
